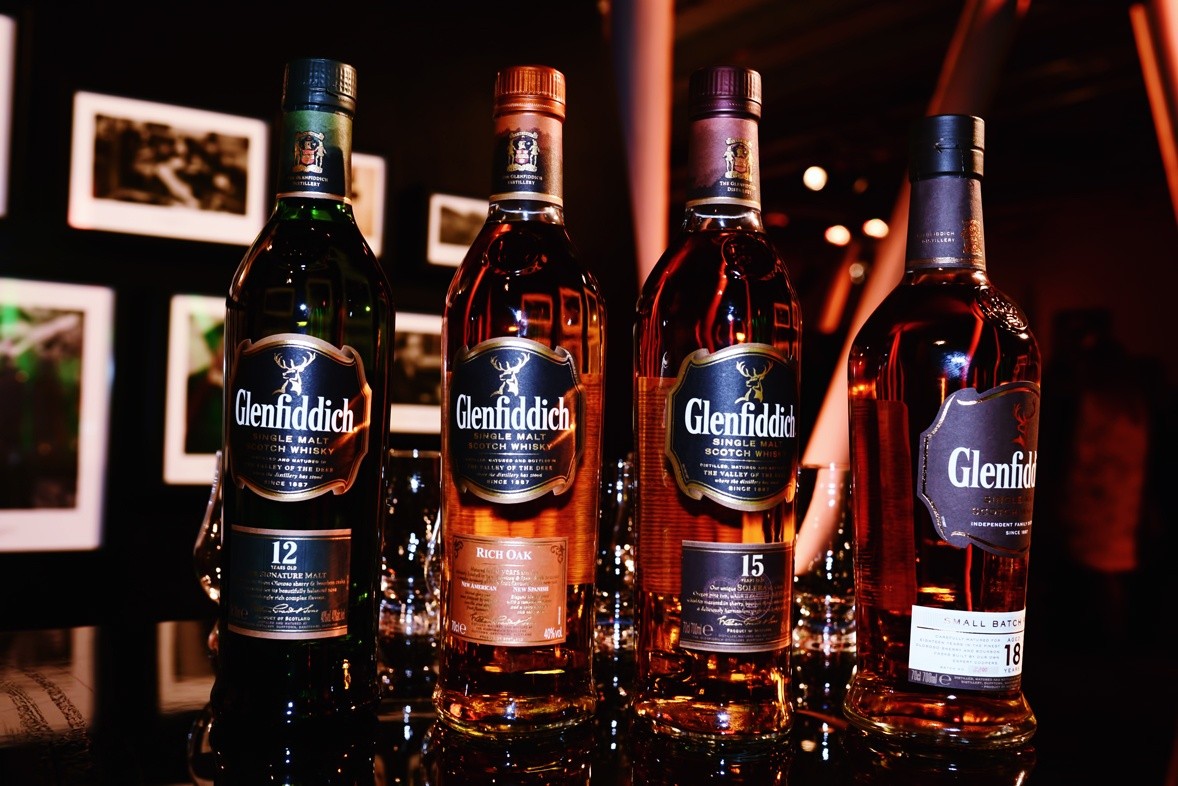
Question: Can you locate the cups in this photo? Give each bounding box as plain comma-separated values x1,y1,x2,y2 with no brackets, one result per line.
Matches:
189,448,853,657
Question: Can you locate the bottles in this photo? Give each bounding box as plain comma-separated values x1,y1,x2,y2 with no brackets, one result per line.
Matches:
847,112,1037,750
433,65,603,740
185,61,386,785
635,69,801,765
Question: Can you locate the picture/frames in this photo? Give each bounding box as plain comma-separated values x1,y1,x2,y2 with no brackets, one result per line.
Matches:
0,278,113,551
67,92,268,245
390,313,443,433
426,194,489,267
163,295,226,484
349,153,386,259
